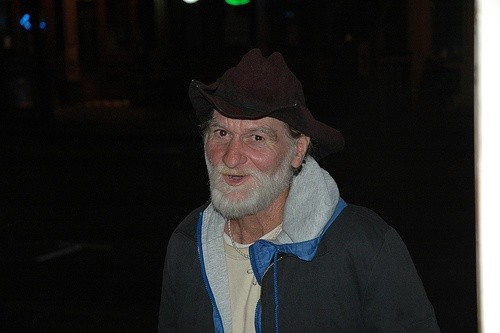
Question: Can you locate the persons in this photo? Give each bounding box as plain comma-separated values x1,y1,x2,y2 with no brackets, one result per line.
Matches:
158,47,442,333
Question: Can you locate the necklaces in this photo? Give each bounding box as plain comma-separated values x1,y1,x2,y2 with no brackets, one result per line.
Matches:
227,218,280,286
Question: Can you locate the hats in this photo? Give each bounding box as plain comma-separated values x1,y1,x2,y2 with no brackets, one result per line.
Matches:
188,48,332,141
